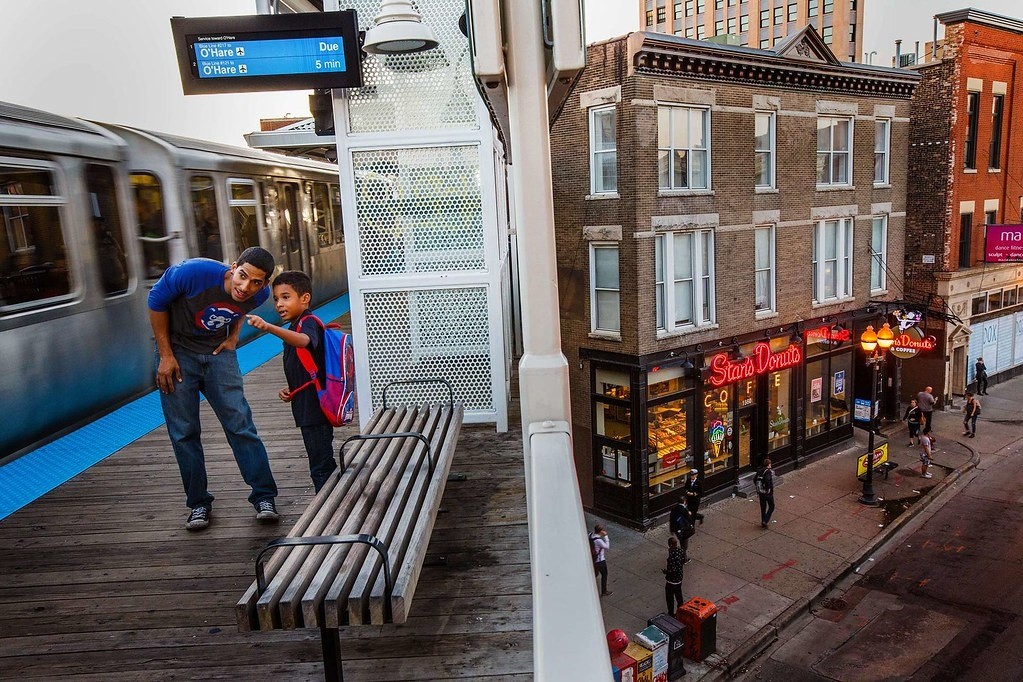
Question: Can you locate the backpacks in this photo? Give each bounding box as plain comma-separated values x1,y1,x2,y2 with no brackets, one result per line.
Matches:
589,532,603,562
287,314,355,427
970,398,981,415
755,468,769,494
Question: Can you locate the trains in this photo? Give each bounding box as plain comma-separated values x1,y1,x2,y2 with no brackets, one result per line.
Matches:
0,102,399,454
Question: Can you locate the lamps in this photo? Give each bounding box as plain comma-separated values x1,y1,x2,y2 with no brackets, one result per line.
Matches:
780,326,802,342
880,307,886,320
822,316,842,330
671,351,694,368
719,341,745,359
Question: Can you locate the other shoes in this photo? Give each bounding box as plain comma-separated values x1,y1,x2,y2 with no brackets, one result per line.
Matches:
963,431,971,436
601,591,613,596
982,391,989,395
685,558,692,565
921,474,932,478
762,521,767,528
917,439,921,446
968,433,975,438
926,471,932,476
977,392,984,396
907,442,914,447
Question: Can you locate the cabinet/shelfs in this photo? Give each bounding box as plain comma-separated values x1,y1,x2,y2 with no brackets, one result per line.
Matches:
648,398,686,459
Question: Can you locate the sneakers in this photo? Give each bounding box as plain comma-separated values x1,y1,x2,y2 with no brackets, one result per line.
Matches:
185,504,212,529
257,502,279,520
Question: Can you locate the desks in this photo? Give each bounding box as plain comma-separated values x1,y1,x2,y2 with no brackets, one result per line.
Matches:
831,411,850,428
648,466,691,493
769,432,788,451
806,419,827,437
704,453,733,473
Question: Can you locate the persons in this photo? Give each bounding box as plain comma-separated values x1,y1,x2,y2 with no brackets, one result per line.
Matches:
919,427,933,479
753,459,775,528
246,271,338,496
93,217,128,294
669,496,691,565
902,398,922,448
588,524,614,596
916,385,938,433
962,393,978,438
147,248,278,531
661,537,685,619
976,357,989,396
685,469,704,529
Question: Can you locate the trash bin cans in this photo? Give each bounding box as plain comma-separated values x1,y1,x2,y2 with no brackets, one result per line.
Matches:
677,598,718,662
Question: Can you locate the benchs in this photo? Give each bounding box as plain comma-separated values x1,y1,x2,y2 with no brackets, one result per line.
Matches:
235,379,463,682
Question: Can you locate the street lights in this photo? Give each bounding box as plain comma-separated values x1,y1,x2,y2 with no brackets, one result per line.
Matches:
857,323,895,506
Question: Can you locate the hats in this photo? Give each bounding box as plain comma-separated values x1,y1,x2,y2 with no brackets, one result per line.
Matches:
690,469,698,475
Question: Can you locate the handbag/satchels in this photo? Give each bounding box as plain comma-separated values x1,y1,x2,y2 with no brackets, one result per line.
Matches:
675,524,696,541
